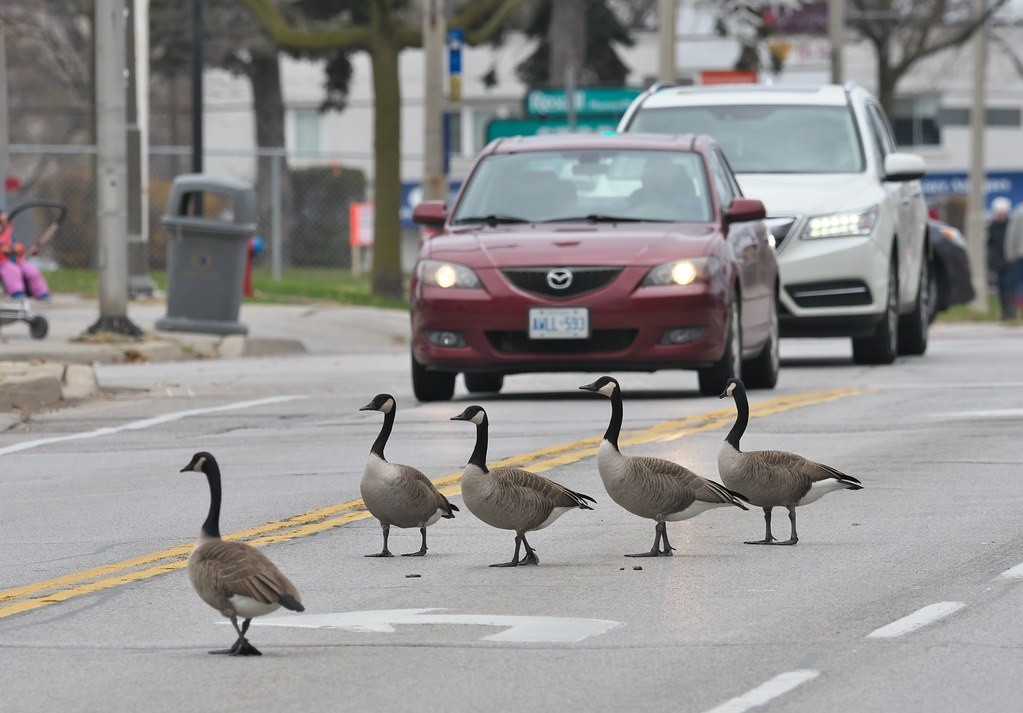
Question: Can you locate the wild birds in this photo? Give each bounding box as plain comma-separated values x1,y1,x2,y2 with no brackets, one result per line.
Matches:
578,375,750,558
716,377,865,546
357,394,460,558
179,451,305,657
449,405,598,568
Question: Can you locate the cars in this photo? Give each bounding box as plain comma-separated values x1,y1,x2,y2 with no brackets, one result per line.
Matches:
404,129,785,396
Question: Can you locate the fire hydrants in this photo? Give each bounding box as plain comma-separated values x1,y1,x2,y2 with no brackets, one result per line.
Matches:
244,232,264,301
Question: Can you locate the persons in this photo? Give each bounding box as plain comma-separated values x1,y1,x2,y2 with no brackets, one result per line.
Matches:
617,155,705,221
0,209,52,303
985,196,1023,321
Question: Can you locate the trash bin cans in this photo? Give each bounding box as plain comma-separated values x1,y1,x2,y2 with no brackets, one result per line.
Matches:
153,175,258,335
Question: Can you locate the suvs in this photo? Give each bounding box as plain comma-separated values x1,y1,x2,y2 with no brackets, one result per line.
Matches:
618,81,932,362
